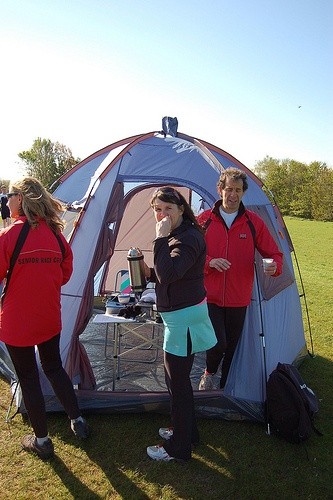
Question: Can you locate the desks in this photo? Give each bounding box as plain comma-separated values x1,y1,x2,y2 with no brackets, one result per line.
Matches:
91,298,164,392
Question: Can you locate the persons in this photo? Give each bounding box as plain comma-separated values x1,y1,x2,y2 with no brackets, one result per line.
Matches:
0,185,10,228
144,187,218,463
0,177,90,458
195,167,284,391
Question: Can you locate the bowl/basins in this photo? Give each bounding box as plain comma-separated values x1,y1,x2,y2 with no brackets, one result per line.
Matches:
118,294,130,304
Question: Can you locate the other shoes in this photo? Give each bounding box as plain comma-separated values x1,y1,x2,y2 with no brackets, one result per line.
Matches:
198,368,216,390
159,427,174,440
71,416,86,436
23,434,54,459
146,445,187,462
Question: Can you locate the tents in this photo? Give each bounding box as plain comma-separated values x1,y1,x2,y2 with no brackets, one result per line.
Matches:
0,117,315,434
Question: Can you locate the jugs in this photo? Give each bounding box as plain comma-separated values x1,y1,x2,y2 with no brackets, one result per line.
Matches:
127,247,147,293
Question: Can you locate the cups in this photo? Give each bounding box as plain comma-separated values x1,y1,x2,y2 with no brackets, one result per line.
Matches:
262,258,274,273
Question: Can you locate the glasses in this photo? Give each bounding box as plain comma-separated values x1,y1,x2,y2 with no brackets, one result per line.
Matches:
6,193,18,199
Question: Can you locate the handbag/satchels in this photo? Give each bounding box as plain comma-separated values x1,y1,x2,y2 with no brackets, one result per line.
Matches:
267,361,324,445
